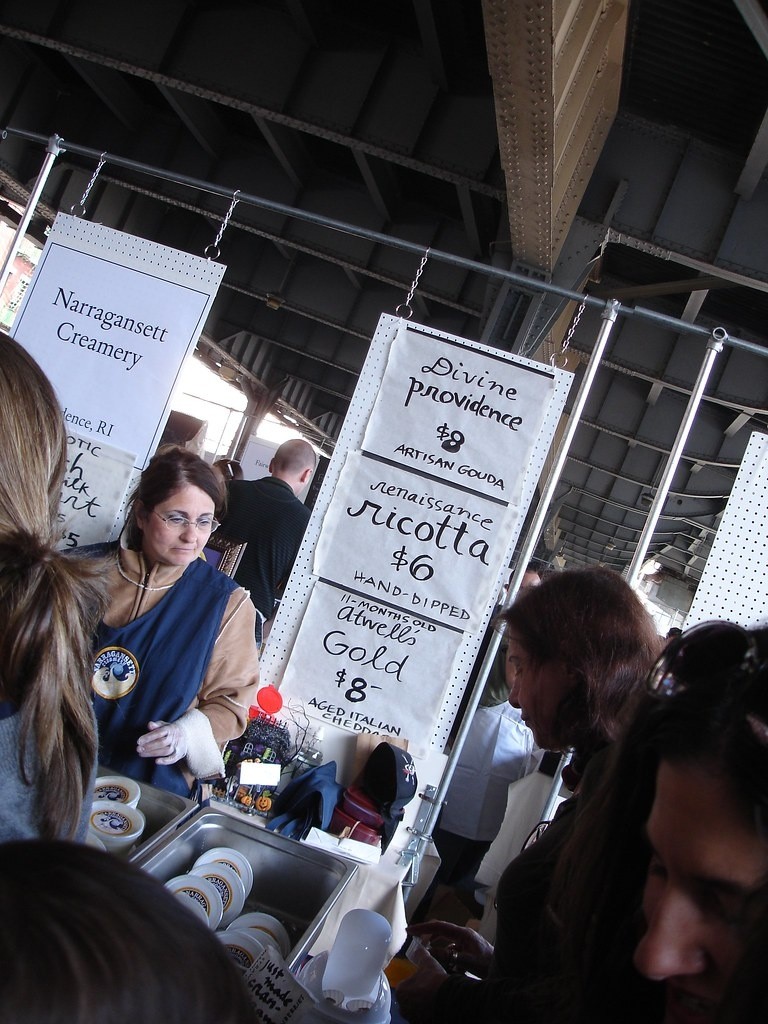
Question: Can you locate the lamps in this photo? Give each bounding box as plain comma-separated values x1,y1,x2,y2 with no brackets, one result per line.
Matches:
266,251,295,309
605,527,620,550
641,456,667,507
598,550,608,567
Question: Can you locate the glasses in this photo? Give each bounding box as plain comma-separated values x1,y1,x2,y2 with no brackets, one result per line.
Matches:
642,621,768,703
152,510,221,534
220,458,234,477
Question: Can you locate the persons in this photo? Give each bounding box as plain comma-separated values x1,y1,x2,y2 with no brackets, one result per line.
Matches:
0,328,317,1024
411,561,767,1023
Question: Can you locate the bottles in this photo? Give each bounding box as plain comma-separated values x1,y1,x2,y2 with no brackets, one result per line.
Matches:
292,726,324,778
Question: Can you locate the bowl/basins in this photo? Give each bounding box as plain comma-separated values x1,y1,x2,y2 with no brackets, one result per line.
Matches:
406,936,432,967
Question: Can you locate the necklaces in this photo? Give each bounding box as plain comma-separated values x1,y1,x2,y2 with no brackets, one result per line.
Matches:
114,552,174,591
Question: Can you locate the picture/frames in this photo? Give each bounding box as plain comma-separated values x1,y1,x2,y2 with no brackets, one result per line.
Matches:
197,531,248,579
